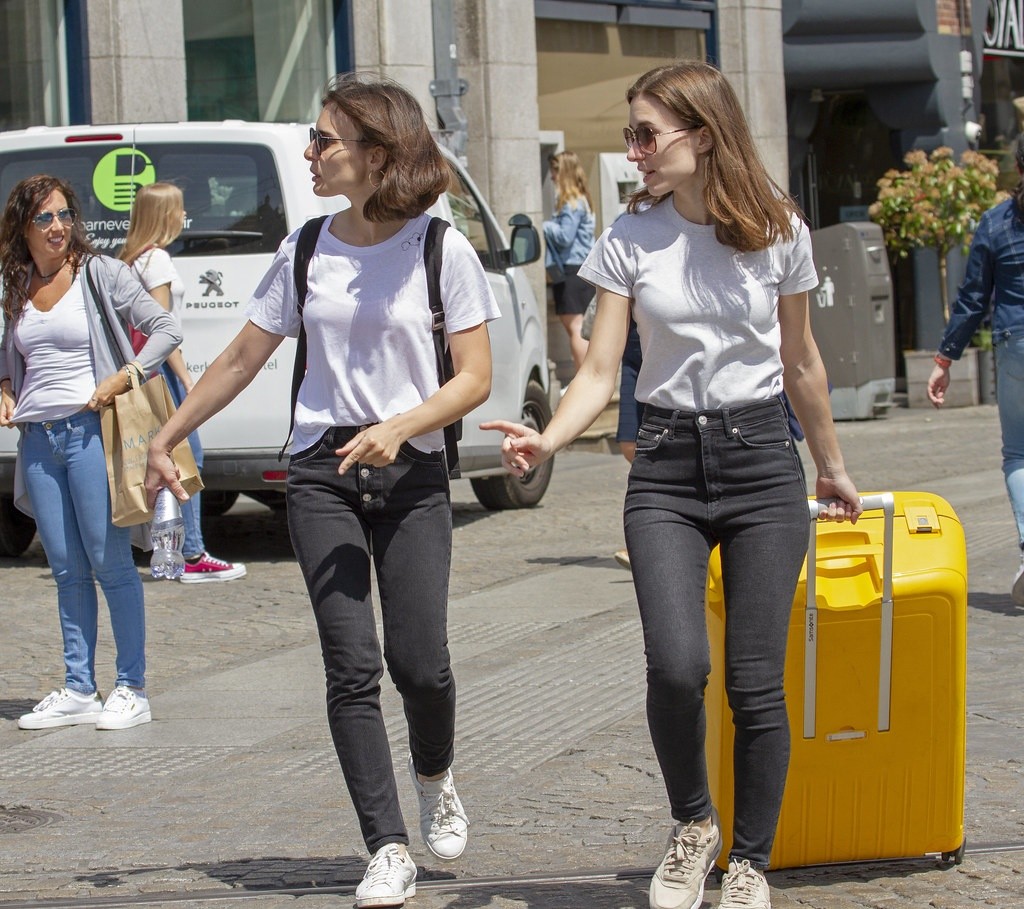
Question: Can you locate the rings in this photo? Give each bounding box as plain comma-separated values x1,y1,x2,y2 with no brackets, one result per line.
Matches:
97,405,103,409
510,461,519,468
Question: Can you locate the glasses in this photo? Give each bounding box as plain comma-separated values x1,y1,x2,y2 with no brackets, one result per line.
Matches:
622,124,703,154
309,127,370,156
30,208,78,228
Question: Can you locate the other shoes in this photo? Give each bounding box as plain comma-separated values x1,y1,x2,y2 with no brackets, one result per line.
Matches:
1010,563,1024,605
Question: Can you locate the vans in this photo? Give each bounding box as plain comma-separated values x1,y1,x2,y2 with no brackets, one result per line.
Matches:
0,118,552,562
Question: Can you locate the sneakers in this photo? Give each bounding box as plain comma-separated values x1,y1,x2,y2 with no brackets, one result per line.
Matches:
717,859,772,909
180,551,247,584
17,688,105,730
407,750,470,860
354,843,418,909
648,805,723,909
96,686,151,730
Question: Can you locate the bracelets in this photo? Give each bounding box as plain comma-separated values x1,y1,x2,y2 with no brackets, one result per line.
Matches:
121,366,133,388
934,353,952,367
0,378,10,384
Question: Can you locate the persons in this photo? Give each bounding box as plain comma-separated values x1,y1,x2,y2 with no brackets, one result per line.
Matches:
610,190,658,569
95,182,247,584
926,130,1024,606
0,174,184,730
141,78,500,909
479,60,864,909
541,149,595,398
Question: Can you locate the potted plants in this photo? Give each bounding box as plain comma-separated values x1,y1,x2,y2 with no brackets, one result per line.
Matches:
869,148,1012,407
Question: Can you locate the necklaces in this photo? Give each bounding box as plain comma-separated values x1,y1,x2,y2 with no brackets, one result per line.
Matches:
34,258,69,285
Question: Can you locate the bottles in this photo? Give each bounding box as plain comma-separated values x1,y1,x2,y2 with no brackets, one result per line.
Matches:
148,486,186,580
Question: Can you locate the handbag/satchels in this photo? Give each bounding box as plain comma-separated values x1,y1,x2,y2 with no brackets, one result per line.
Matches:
98,361,205,528
543,233,568,289
